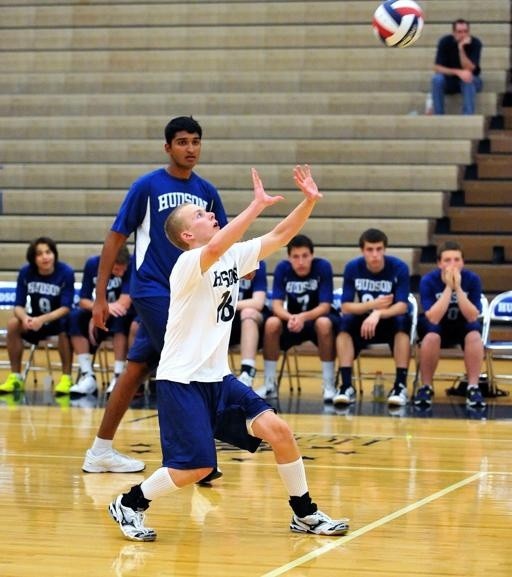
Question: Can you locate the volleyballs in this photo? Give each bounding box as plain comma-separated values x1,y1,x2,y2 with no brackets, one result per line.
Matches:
371,0,424,49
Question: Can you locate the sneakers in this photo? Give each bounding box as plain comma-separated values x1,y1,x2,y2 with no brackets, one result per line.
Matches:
322,385,336,401
103,373,119,392
81,448,147,474
289,509,350,537
234,369,253,389
413,386,434,406
51,375,73,394
387,382,409,406
333,387,357,407
106,492,158,541
68,373,98,397
254,383,279,401
0,371,26,392
465,386,487,408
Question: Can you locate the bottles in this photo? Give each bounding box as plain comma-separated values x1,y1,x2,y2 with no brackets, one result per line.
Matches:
424,92,434,114
374,371,384,399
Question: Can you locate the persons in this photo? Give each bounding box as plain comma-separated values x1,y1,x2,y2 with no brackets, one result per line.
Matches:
256,234,338,403
107,163,349,543
82,116,229,484
228,260,272,389
431,19,483,115
0,237,75,394
332,228,414,407
413,241,487,408
66,244,132,396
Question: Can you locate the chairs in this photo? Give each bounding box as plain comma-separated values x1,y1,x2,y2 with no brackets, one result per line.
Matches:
351,292,417,393
229,291,293,392
278,288,343,393
411,292,491,399
24,281,105,386
483,290,512,395
91,335,156,395
0,280,38,383
